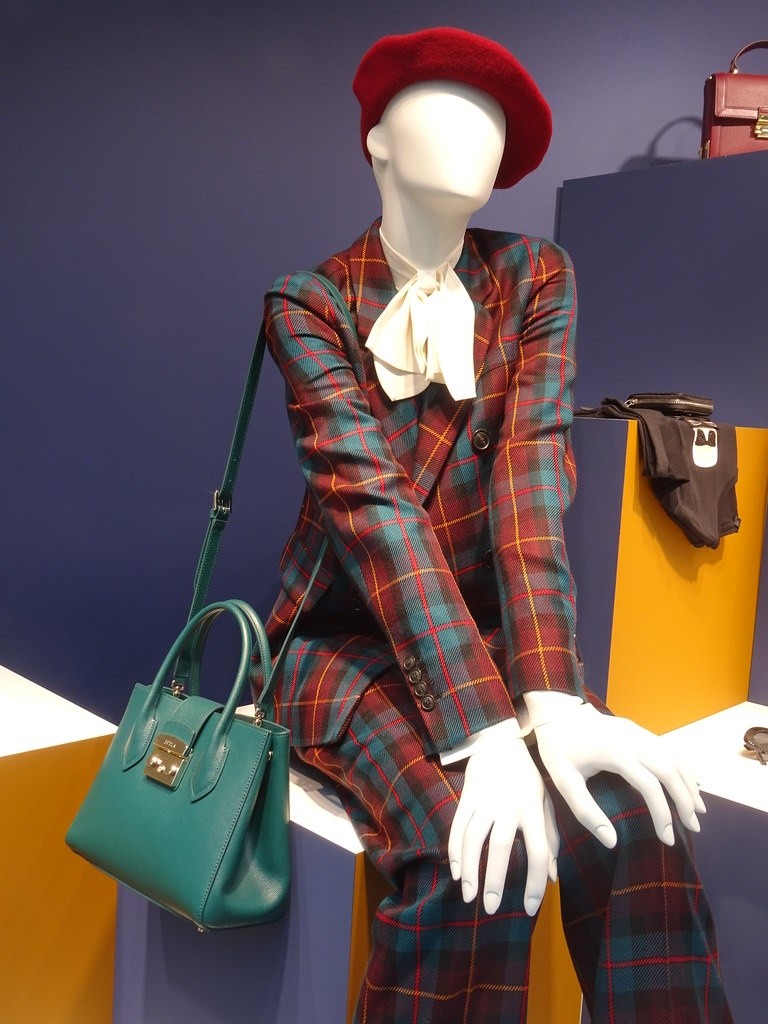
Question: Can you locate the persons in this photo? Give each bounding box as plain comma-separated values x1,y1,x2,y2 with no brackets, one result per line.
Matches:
245,29,734,1024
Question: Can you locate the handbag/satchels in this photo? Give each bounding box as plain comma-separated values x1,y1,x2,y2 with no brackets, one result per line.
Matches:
699,40,768,158
65,599,294,933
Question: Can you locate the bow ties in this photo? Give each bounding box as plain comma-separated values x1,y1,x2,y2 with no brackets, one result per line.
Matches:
363,229,477,402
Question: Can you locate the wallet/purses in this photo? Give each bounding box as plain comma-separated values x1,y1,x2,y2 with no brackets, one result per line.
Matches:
624,391,713,418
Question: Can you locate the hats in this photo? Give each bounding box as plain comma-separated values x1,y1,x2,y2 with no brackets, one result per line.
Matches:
354,26,553,189
